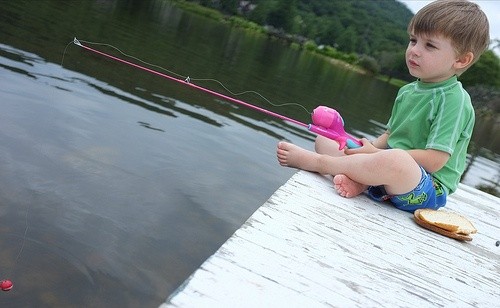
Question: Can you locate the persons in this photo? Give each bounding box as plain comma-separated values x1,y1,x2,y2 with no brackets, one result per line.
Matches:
276,0,490,214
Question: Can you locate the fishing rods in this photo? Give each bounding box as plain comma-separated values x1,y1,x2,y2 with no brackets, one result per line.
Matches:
71,37,363,150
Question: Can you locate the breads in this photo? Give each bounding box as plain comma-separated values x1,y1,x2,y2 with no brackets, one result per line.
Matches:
414,208,477,241
